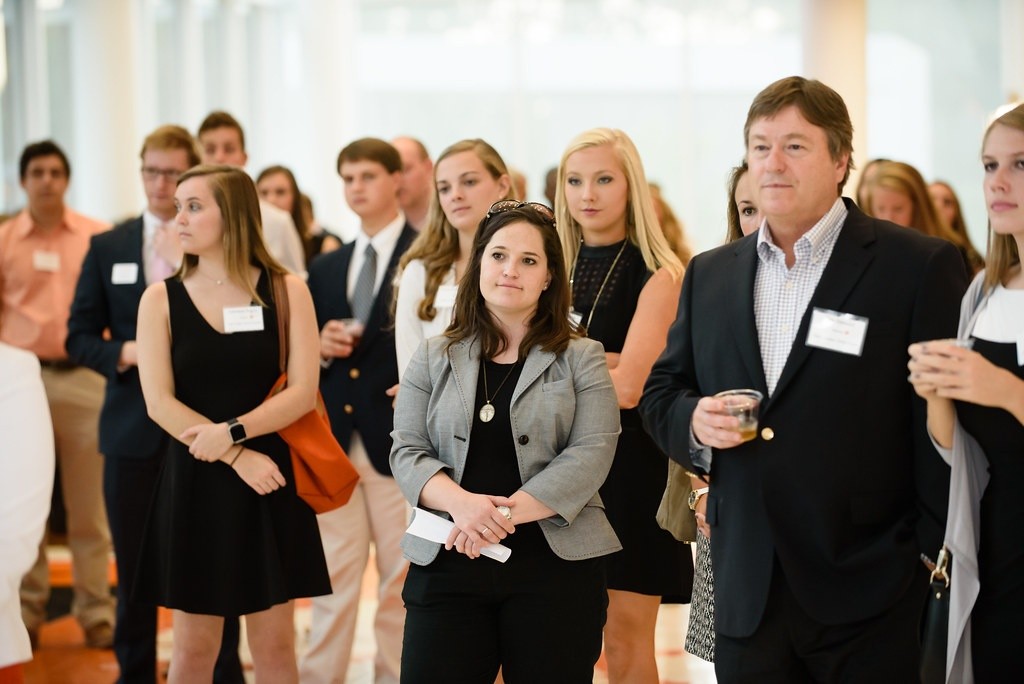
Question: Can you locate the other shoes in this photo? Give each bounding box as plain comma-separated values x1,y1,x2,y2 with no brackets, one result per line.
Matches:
28,629,38,650
40,582,75,625
86,622,116,648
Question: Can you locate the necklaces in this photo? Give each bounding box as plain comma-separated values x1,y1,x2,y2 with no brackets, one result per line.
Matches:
479,354,518,422
194,267,230,285
569,234,629,336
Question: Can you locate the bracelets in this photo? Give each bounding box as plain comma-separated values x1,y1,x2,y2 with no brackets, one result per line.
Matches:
229,447,245,467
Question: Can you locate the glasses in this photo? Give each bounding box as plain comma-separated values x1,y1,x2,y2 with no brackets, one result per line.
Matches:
143,166,184,183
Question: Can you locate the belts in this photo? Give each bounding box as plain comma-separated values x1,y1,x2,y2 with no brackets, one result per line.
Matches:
40,359,85,371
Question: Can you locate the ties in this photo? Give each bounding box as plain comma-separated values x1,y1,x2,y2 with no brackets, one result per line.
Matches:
352,242,378,325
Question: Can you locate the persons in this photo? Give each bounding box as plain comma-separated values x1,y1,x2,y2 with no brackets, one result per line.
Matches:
0,137,124,650
0,338,56,684
255,165,343,255
388,197,622,684
295,138,425,684
63,125,247,684
639,76,982,684
544,128,694,684
383,138,435,232
386,138,527,409
199,112,309,286
135,167,320,684
907,104,1024,684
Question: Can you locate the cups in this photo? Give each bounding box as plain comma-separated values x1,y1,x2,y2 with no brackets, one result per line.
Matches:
714,390,763,441
338,321,362,347
924,340,975,389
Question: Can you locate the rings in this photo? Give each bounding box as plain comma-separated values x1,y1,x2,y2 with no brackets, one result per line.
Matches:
481,528,488,534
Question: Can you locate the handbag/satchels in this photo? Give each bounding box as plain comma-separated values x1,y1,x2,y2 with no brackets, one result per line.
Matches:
913,575,952,684
263,268,359,513
656,458,696,541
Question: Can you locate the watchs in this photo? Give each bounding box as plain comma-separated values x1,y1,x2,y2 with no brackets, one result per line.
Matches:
496,506,511,521
688,486,709,510
227,417,247,443
